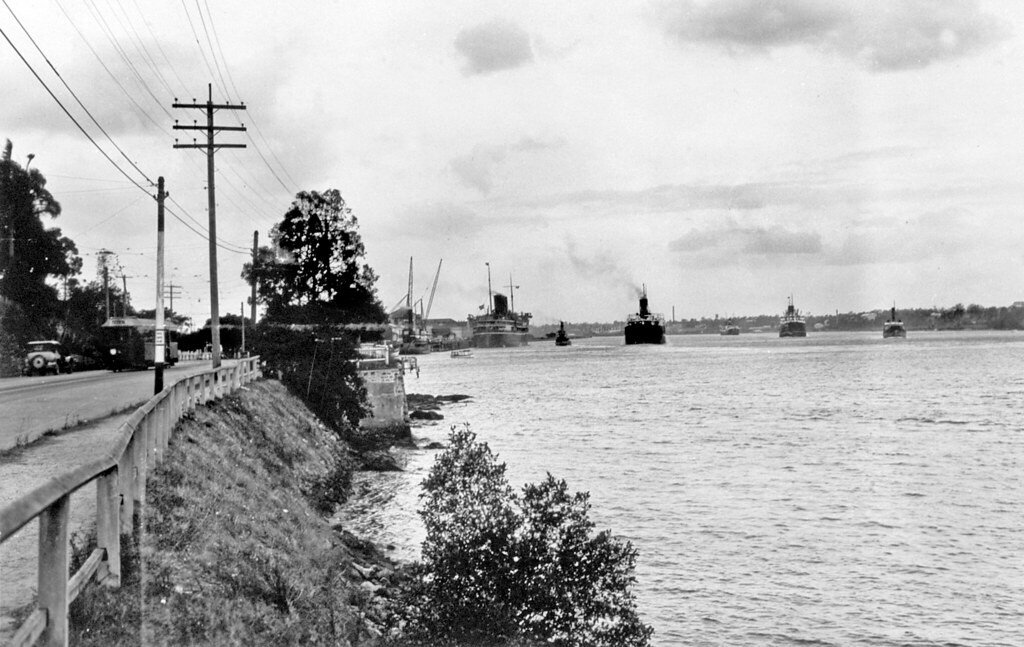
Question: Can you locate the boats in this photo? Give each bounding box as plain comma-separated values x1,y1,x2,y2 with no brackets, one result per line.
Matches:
555,321,572,348
466,261,533,347
623,282,667,344
778,291,806,337
882,303,906,339
717,317,740,335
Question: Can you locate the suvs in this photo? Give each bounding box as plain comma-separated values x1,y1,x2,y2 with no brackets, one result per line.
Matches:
22,340,75,376
